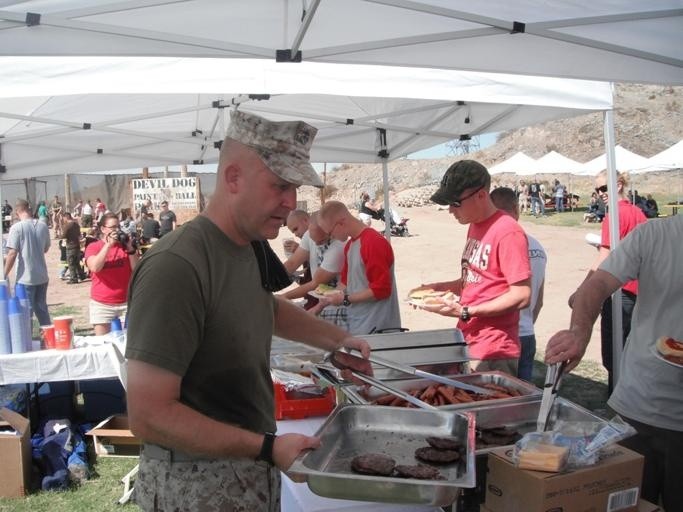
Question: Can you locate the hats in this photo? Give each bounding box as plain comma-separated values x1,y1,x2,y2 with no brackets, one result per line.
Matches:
227,110,324,188
430,160,487,205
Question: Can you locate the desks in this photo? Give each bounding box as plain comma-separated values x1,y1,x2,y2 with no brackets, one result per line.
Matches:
664,204,683,214
1,328,128,418
271,334,665,511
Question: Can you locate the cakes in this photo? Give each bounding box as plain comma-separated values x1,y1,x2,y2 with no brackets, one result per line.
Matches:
515,442,572,473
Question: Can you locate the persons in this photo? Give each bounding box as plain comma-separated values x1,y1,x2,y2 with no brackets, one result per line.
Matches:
552,181,566,213
0,195,177,337
406,160,533,378
488,186,547,381
634,190,639,204
647,192,659,212
517,180,549,218
276,191,409,336
625,190,633,204
119,109,373,512
545,212,683,512
583,192,606,225
567,166,648,402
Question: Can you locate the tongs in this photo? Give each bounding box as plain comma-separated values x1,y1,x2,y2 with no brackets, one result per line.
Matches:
537,361,571,433
329,348,500,408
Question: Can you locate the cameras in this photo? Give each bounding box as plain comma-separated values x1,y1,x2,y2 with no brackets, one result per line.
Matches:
111,231,130,245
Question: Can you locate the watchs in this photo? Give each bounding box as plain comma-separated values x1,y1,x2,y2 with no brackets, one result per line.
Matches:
254,430,278,470
460,306,470,321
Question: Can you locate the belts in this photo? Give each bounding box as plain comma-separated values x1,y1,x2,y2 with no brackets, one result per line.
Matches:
144,443,200,462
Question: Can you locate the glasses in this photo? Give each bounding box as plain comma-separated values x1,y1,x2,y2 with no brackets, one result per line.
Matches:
103,225,120,228
329,223,337,236
452,185,485,207
595,185,607,194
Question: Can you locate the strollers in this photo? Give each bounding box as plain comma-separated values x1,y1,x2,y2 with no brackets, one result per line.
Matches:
378,206,410,239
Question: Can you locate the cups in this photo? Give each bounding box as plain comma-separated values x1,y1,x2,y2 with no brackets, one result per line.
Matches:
282,238,295,257
39,325,55,350
53,316,73,350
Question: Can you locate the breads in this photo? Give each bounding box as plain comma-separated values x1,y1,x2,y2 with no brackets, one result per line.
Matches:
397,463,439,480
414,287,457,307
415,444,460,466
425,434,461,452
483,429,519,446
313,282,340,297
348,451,395,476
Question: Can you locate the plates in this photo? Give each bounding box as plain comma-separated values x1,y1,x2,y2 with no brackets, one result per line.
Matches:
409,294,459,307
649,345,682,369
307,290,344,298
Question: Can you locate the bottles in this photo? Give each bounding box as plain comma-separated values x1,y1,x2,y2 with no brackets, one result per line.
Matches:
7,297,26,353
0,285,11,353
111,319,124,341
15,284,32,350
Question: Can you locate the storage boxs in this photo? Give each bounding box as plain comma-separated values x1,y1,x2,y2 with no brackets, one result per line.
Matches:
486,444,644,512
0,407,31,497
85,412,144,458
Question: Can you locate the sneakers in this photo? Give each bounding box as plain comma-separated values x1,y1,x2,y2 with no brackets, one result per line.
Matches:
67,279,78,284
79,274,86,279
